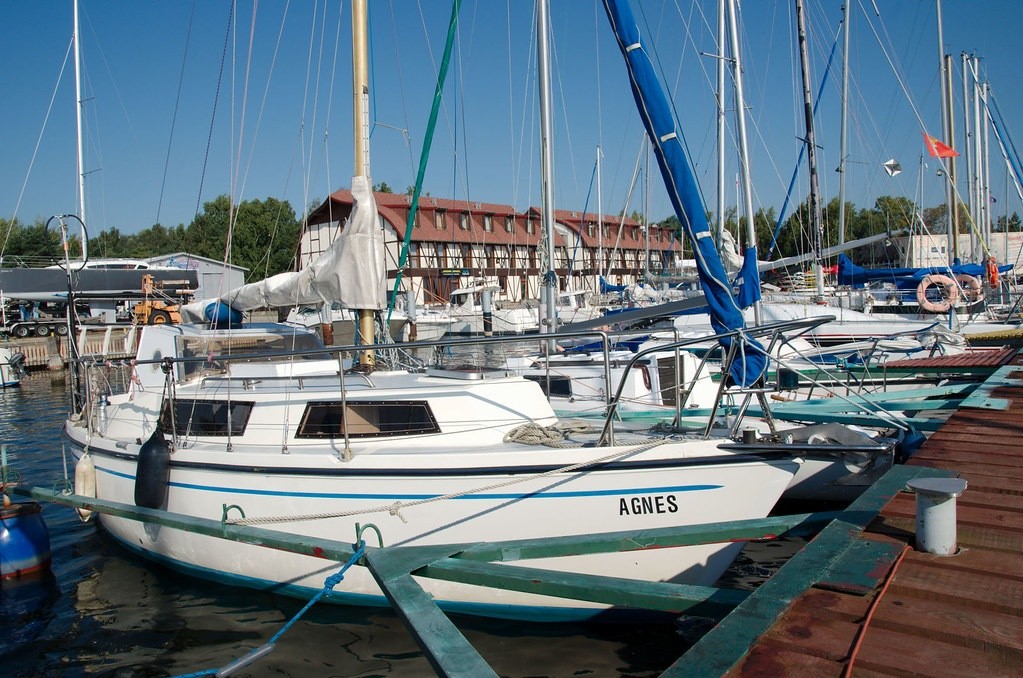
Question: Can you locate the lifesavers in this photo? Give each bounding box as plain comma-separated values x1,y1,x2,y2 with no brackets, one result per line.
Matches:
916,275,958,312
986,256,999,289
955,275,980,301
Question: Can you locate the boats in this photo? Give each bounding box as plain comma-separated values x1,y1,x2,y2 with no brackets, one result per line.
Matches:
0,501,55,582
0,347,28,389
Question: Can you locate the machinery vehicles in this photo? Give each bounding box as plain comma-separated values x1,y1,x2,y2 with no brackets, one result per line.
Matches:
1,295,108,337
133,275,196,326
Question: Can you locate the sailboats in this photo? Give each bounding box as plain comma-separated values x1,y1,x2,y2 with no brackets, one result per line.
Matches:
1,1,1023,624
